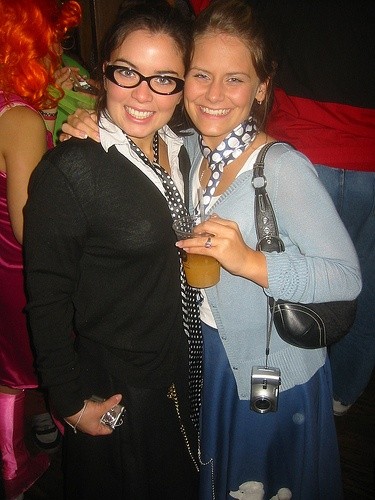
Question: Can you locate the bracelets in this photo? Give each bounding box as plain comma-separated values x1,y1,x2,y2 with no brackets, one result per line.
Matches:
40,111,58,116
64,402,88,434
44,116,57,120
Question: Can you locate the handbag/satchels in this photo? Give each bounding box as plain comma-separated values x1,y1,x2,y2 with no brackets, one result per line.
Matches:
250,138,358,350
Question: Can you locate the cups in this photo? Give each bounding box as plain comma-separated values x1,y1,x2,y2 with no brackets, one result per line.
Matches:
172,214,221,290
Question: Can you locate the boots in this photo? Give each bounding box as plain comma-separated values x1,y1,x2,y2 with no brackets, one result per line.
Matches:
0,390,54,500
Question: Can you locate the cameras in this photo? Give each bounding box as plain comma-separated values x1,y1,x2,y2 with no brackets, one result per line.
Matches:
250,366,281,414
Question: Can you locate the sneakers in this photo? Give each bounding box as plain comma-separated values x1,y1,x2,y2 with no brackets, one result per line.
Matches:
30,410,63,455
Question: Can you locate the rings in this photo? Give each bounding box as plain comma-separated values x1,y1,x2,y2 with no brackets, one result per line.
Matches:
205,237,211,248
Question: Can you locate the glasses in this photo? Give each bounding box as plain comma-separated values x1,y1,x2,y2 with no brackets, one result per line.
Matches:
104,64,185,96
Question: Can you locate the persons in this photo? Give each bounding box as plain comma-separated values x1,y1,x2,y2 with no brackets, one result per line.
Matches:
59,0,362,500
23,0,204,500
0,0,102,500
263,0,375,416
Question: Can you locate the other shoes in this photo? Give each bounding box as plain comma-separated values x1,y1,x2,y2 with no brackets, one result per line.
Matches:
333,392,362,417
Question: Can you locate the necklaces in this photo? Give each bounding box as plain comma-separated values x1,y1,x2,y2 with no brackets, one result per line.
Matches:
200,170,206,181
